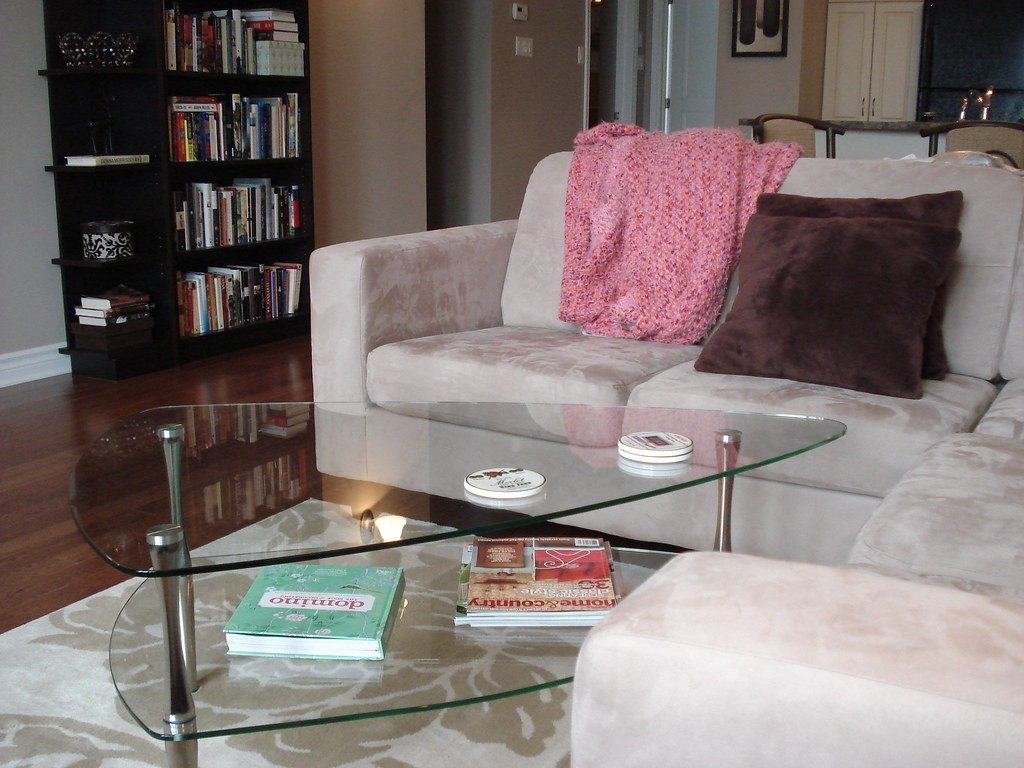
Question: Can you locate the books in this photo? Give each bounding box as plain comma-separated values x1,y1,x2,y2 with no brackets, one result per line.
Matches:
166,92,300,162
165,1,299,75
453,533,622,628
222,563,407,661
175,261,303,336
183,403,310,526
74,283,151,326
64,152,150,166
170,177,301,251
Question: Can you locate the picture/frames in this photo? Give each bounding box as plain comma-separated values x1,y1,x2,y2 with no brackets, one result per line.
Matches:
731,0,791,59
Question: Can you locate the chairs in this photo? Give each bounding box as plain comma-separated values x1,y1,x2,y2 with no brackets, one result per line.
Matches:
737,112,848,160
918,112,1024,174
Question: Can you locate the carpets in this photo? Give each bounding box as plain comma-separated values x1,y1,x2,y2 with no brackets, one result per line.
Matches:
0,496,660,768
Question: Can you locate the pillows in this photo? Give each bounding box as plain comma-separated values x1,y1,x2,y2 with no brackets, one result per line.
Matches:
755,190,965,381
695,214,962,402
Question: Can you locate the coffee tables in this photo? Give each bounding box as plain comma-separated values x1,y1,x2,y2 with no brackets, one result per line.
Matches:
71,404,847,768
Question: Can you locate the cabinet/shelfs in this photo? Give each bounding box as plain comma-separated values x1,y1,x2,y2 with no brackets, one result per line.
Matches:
37,0,315,384
823,0,928,122
172,403,326,547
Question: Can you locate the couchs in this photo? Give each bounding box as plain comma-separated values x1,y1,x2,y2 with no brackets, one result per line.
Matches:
309,152,1024,567
568,434,1024,768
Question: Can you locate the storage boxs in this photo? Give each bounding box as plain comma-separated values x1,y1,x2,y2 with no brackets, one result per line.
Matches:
67,321,155,352
